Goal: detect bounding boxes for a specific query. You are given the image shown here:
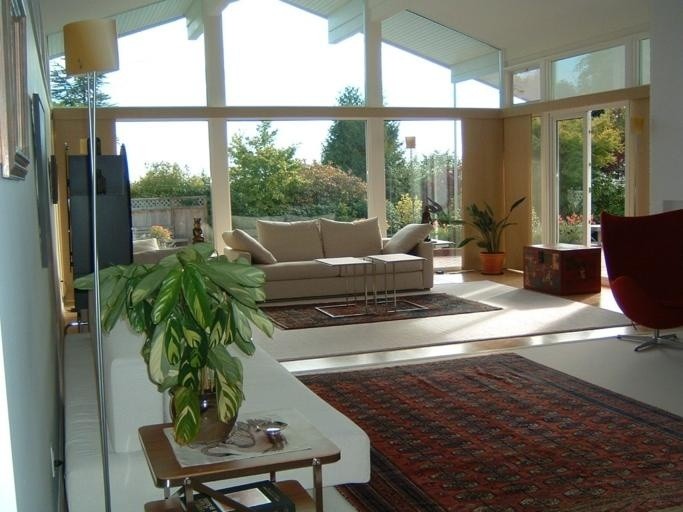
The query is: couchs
[56,266,373,510]
[221,240,435,300]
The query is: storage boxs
[523,243,602,293]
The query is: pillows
[222,228,280,265]
[132,235,157,253]
[256,216,325,267]
[382,222,434,257]
[318,216,385,257]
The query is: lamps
[404,134,420,221]
[61,17,131,512]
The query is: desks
[136,411,340,512]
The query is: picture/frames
[2,0,53,272]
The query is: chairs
[598,206,683,352]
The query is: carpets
[225,277,637,362]
[293,351,682,511]
[254,291,505,332]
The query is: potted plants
[69,240,272,445]
[428,197,533,274]
